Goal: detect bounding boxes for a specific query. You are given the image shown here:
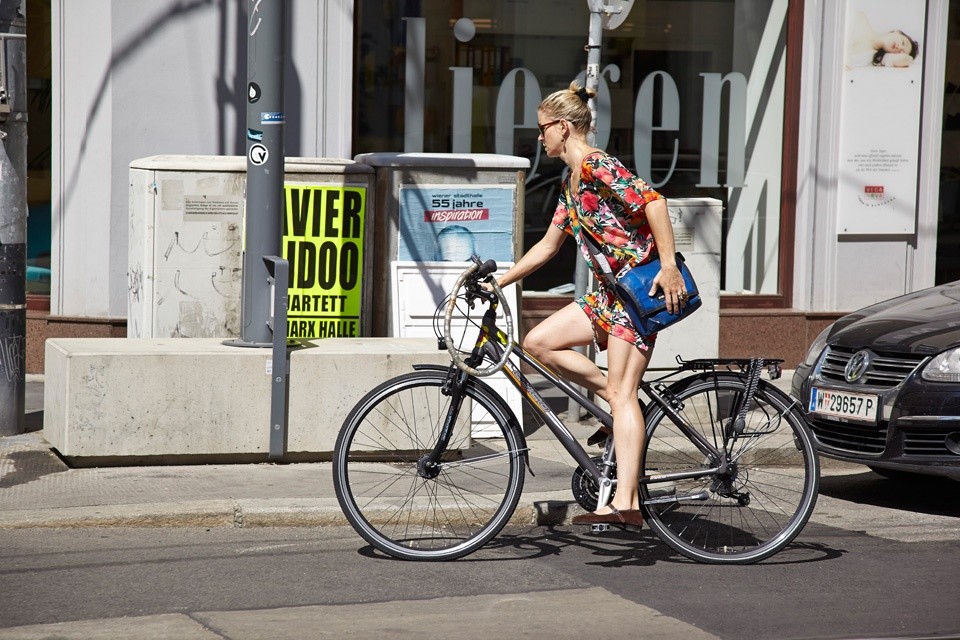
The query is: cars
[794,282,960,475]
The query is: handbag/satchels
[616,253,702,338]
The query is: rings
[679,297,684,301]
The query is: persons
[845,19,919,71]
[485,80,688,534]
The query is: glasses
[537,115,576,139]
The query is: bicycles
[331,253,822,565]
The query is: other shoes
[585,397,646,446]
[572,503,643,533]
